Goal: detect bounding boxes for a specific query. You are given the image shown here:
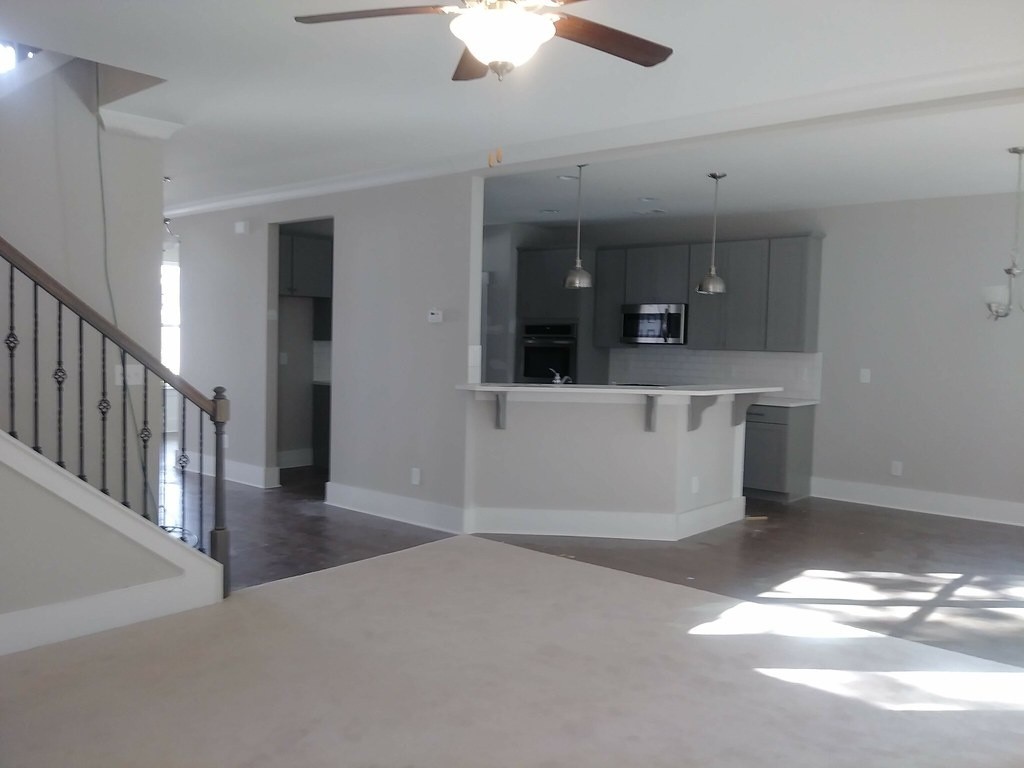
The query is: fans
[294,0,674,80]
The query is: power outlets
[410,467,421,485]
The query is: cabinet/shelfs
[279,230,333,299]
[742,406,814,505]
[593,231,826,353]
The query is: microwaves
[620,302,686,346]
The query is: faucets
[549,366,573,384]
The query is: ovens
[519,318,577,384]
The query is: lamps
[449,7,555,82]
[564,165,593,290]
[694,172,726,294]
